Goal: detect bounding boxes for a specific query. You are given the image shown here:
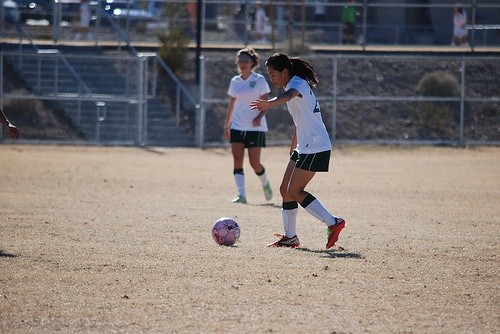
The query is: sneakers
[267,234,300,248]
[231,196,246,203]
[326,218,346,249]
[262,181,273,201]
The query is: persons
[0,106,20,140]
[452,5,470,48]
[224,48,273,204]
[340,1,360,45]
[0,0,270,47]
[250,53,346,249]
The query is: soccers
[210,216,242,245]
